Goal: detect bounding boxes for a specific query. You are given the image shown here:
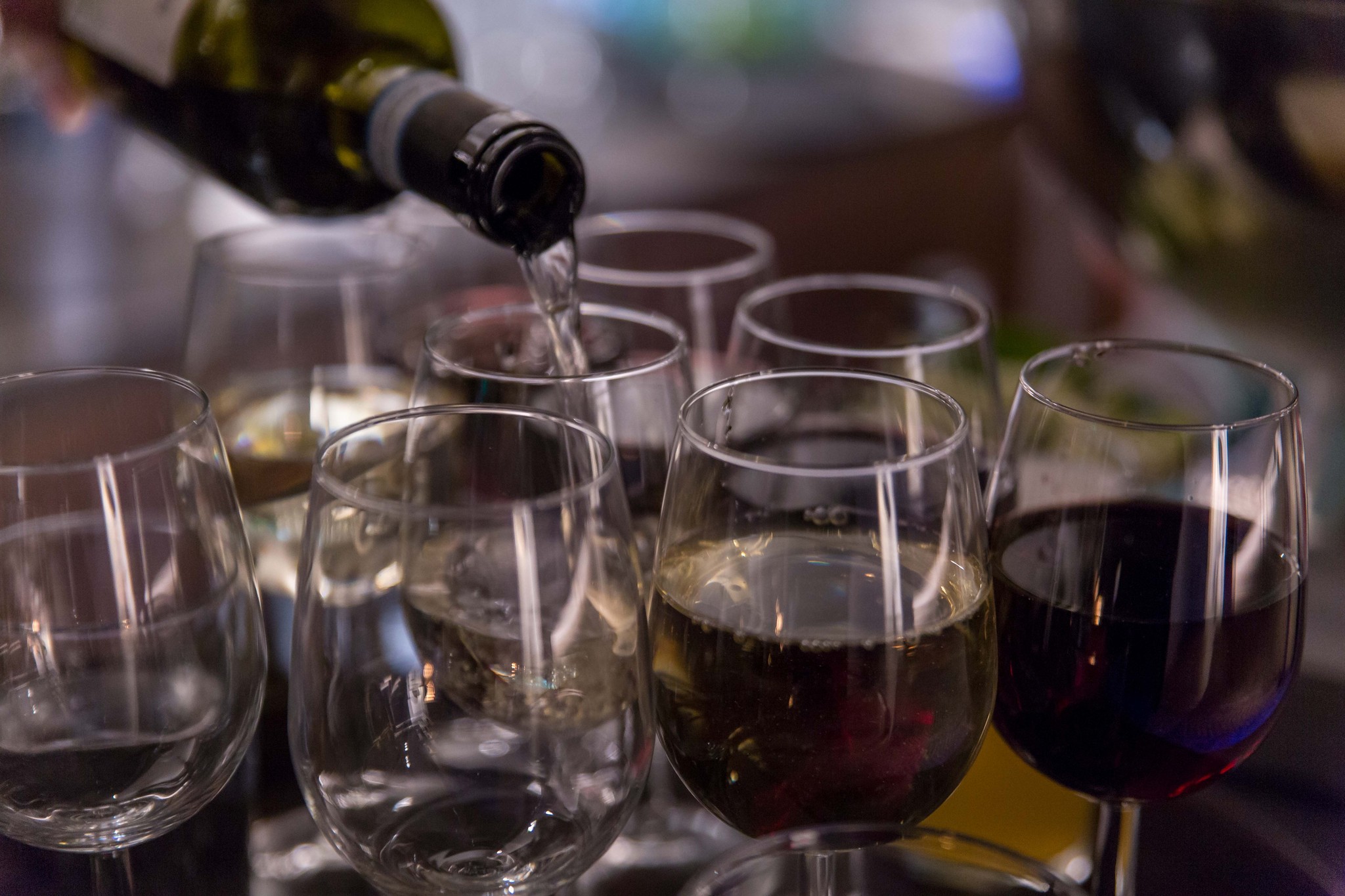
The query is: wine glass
[4,198,1309,896]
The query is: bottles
[0,2,592,251]
